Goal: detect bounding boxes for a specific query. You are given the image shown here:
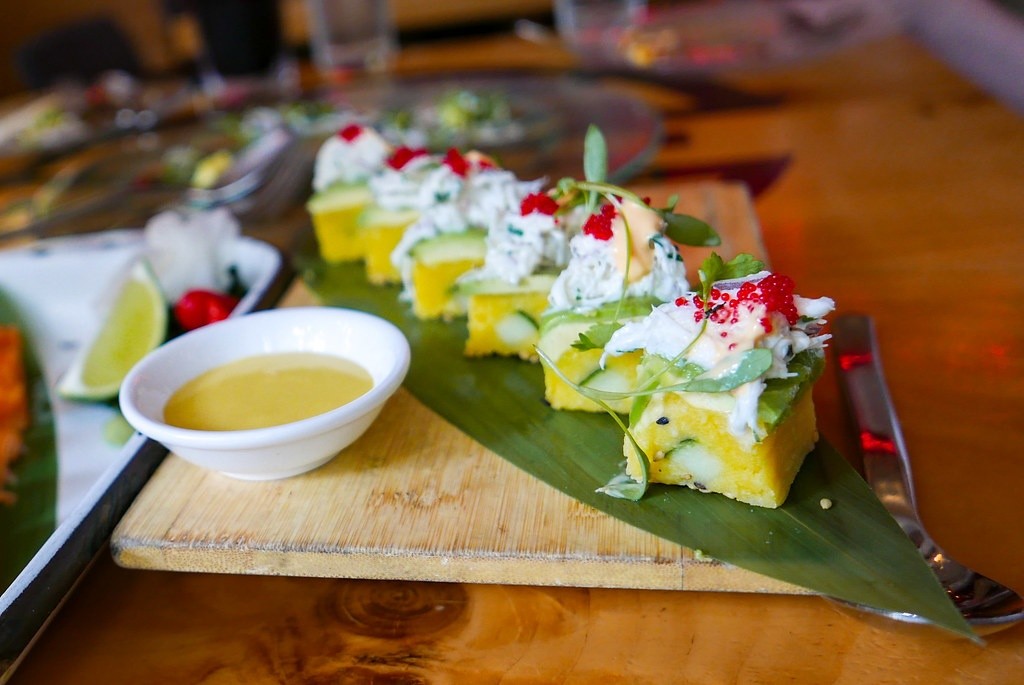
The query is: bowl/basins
[118,306,413,482]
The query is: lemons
[59,260,168,400]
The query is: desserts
[303,122,837,510]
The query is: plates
[0,227,285,685]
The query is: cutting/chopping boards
[108,178,842,596]
